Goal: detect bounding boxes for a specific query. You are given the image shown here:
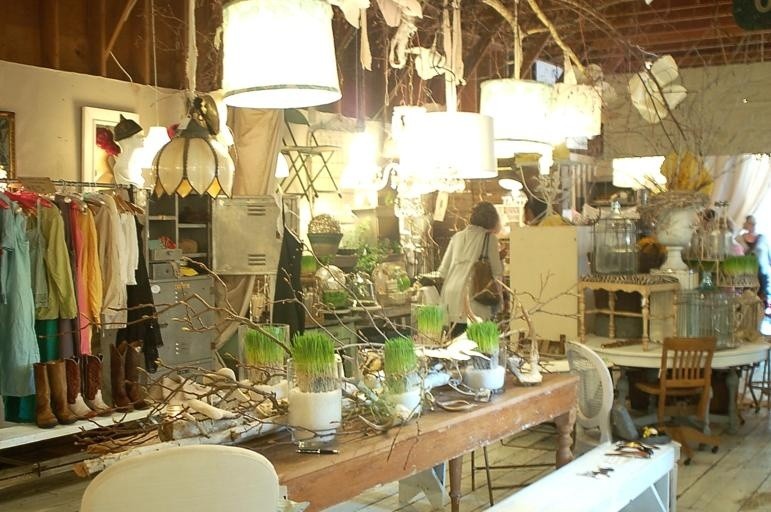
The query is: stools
[577,273,682,352]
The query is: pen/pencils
[295,449,340,454]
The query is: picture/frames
[0,110,17,180]
[79,106,141,192]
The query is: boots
[84,352,113,416]
[66,356,98,420]
[126,337,152,410]
[33,362,60,428]
[46,360,79,425]
[110,340,134,413]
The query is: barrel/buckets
[688,268,727,350]
[593,198,638,274]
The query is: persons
[436,200,504,335]
[735,214,771,318]
[114,114,145,189]
[696,207,717,236]
[522,196,547,226]
[716,216,745,255]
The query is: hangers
[1,178,146,215]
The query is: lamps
[212,1,343,110]
[131,0,238,200]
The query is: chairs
[633,334,722,466]
[564,340,614,460]
[278,111,344,203]
[78,443,280,511]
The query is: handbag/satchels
[473,261,501,306]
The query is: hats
[114,113,144,142]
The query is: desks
[234,371,583,512]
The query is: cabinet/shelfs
[126,189,280,388]
